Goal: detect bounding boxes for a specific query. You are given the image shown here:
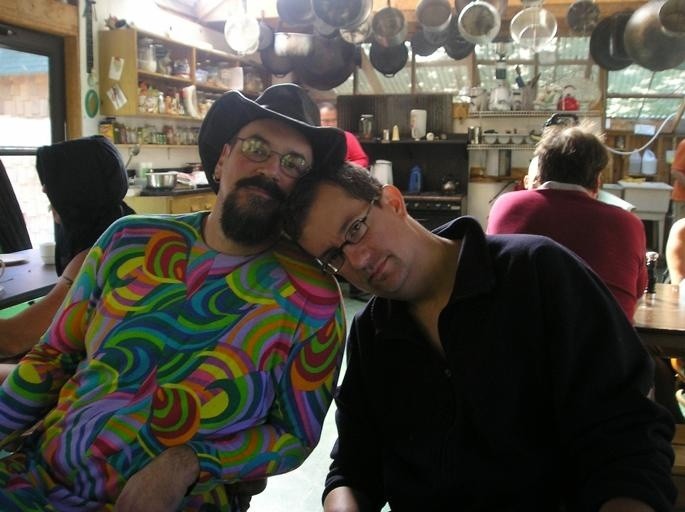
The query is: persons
[315,102,374,302]
[284,160,678,512]
[666,138,685,288]
[0,134,138,357]
[0,83,349,512]
[524,155,637,215]
[485,116,648,329]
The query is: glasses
[230,135,313,180]
[320,196,379,274]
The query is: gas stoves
[402,190,465,199]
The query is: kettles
[374,160,394,186]
[356,114,376,139]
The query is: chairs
[223,477,266,512]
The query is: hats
[197,84,346,197]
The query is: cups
[40,243,56,265]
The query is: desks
[617,180,674,257]
[0,249,62,311]
[633,281,685,356]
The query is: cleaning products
[145,162,153,173]
[136,163,146,177]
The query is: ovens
[407,209,461,231]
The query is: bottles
[511,92,522,111]
[138,38,156,72]
[629,147,641,174]
[411,109,427,141]
[107,118,121,144]
[392,125,400,141]
[409,165,424,193]
[645,252,659,293]
[137,128,143,144]
[99,122,114,144]
[383,129,389,141]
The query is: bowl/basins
[145,172,177,189]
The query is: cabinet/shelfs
[98,29,271,121]
[465,108,604,151]
[123,190,219,215]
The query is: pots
[259,9,275,52]
[416,1,452,32]
[445,16,475,60]
[261,52,294,79]
[372,0,409,46]
[509,0,558,51]
[411,30,439,56]
[295,34,353,90]
[224,0,260,52]
[370,44,409,77]
[277,0,315,23]
[590,10,634,71]
[457,0,502,45]
[624,0,685,71]
[566,1,599,35]
[312,0,372,29]
[339,15,371,44]
[274,32,316,58]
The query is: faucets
[125,153,137,170]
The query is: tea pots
[440,176,460,195]
[558,85,580,110]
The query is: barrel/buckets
[641,148,657,176]
[628,147,641,177]
